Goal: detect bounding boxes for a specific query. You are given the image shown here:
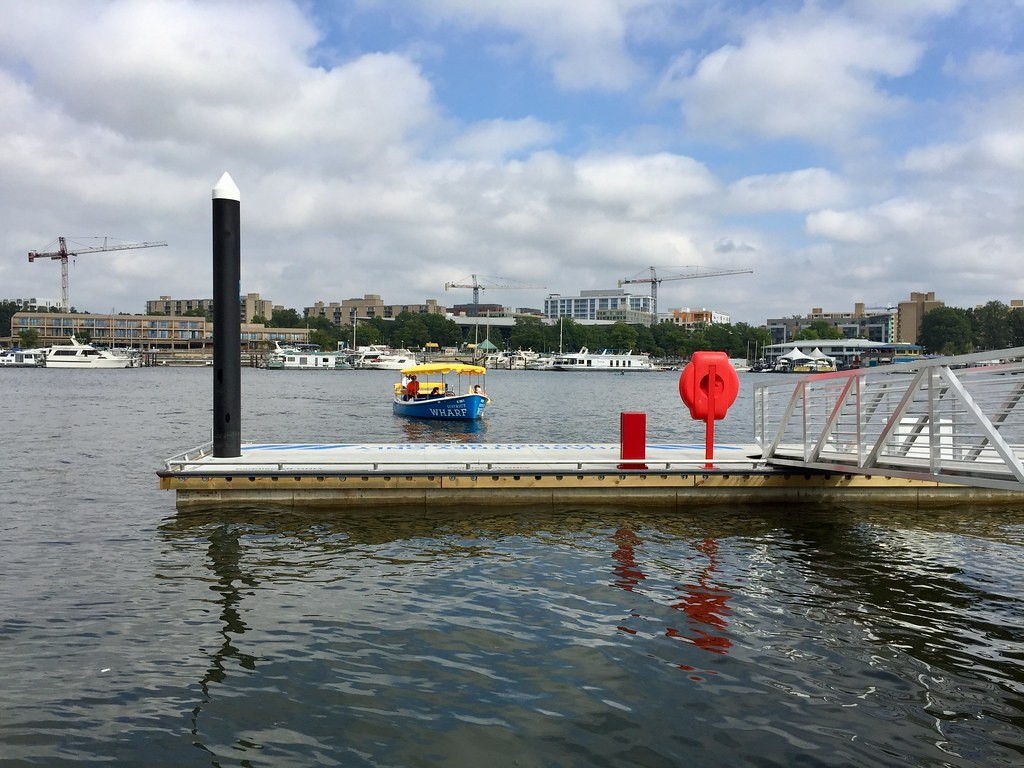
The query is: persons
[406,375,419,401]
[474,385,480,393]
[402,374,412,389]
[429,387,442,398]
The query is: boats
[393,363,491,418]
[728,359,751,372]
[370,355,417,369]
[484,346,563,371]
[860,343,929,375]
[45,335,132,368]
[553,347,667,372]
[0,347,52,368]
[105,348,144,367]
[346,347,382,370]
[256,341,355,371]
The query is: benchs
[394,382,445,401]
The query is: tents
[477,339,498,358]
[777,347,814,372]
[800,347,837,371]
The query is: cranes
[445,273,547,311]
[618,265,753,324]
[28,235,168,311]
